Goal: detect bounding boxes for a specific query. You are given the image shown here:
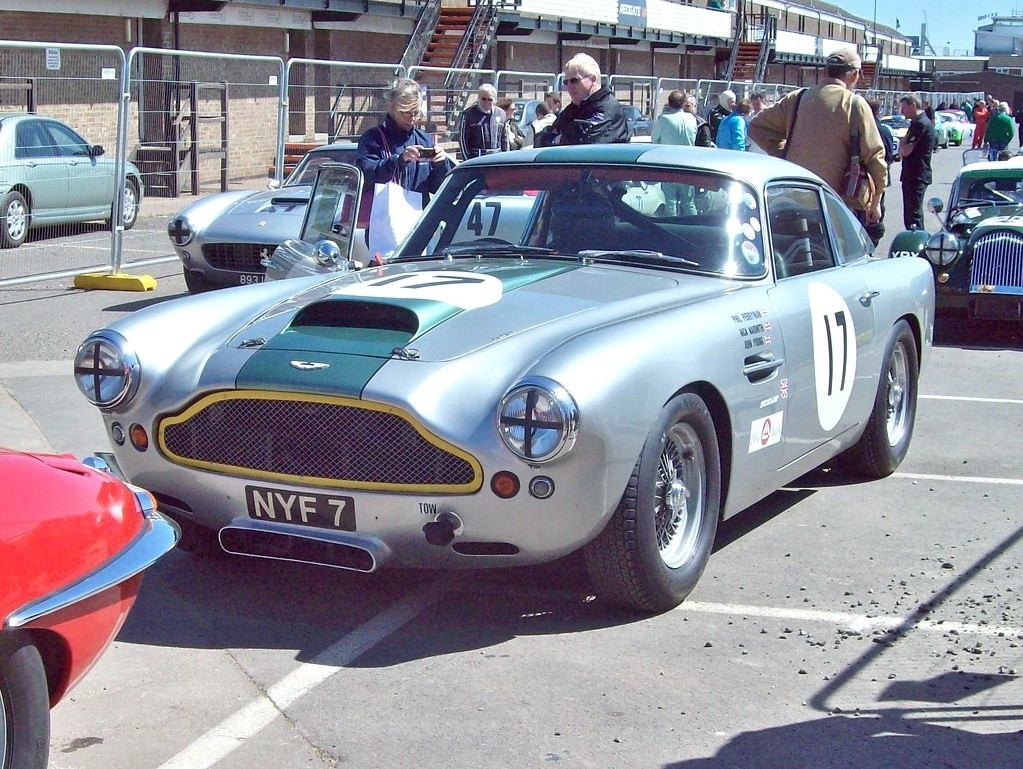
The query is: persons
[355,78,452,255]
[970,95,1015,162]
[459,83,510,160]
[532,52,630,241]
[706,91,737,146]
[704,95,720,121]
[865,98,894,223]
[899,95,933,229]
[747,93,767,155]
[522,102,561,147]
[1015,103,1023,147]
[938,98,973,119]
[546,91,563,118]
[683,93,710,147]
[748,48,888,245]
[648,89,697,216]
[924,100,940,153]
[716,99,752,151]
[494,98,526,151]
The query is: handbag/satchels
[339,128,399,228]
[368,164,423,261]
[839,163,876,211]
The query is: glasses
[564,74,593,86]
[480,96,493,102]
[506,108,516,113]
[395,108,423,119]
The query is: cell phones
[417,148,437,159]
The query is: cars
[510,99,548,140]
[888,147,1022,330]
[0,112,145,251]
[621,104,652,140]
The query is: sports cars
[72,140,939,611]
[166,138,674,298]
[880,108,973,163]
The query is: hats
[827,48,865,80]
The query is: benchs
[614,213,818,278]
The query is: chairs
[543,192,616,258]
